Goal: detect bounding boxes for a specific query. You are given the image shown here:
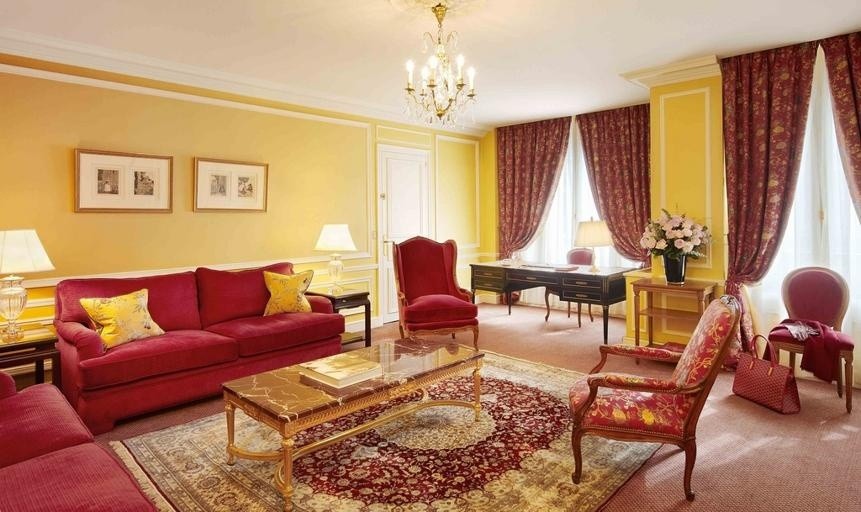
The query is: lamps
[0,229,56,343]
[575,215,614,273]
[312,224,356,292]
[390,1,478,129]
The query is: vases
[663,254,687,286]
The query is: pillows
[196,262,294,329]
[262,270,313,316]
[76,289,166,350]
[59,272,201,331]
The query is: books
[299,354,382,389]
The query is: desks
[470,264,643,345]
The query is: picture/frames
[75,148,173,214]
[194,158,269,213]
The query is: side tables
[0,326,62,392]
[630,278,717,349]
[310,290,371,347]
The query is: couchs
[0,370,160,512]
[52,281,345,436]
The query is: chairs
[570,295,742,501]
[545,247,595,328]
[768,266,854,414]
[393,236,479,350]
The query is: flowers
[640,208,712,259]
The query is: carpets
[108,343,663,512]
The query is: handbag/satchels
[732,335,802,415]
